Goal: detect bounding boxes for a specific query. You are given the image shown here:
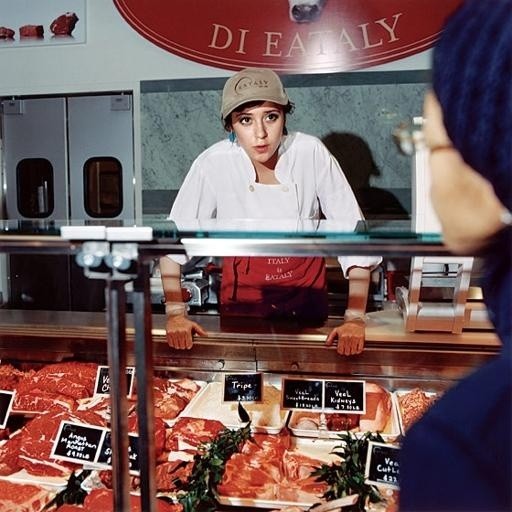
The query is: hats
[431,1,512,212]
[222,68,288,118]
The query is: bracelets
[343,309,370,325]
[164,301,191,316]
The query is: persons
[396,0,511,512]
[159,68,384,357]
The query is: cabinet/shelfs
[1,227,504,512]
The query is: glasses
[392,117,456,157]
